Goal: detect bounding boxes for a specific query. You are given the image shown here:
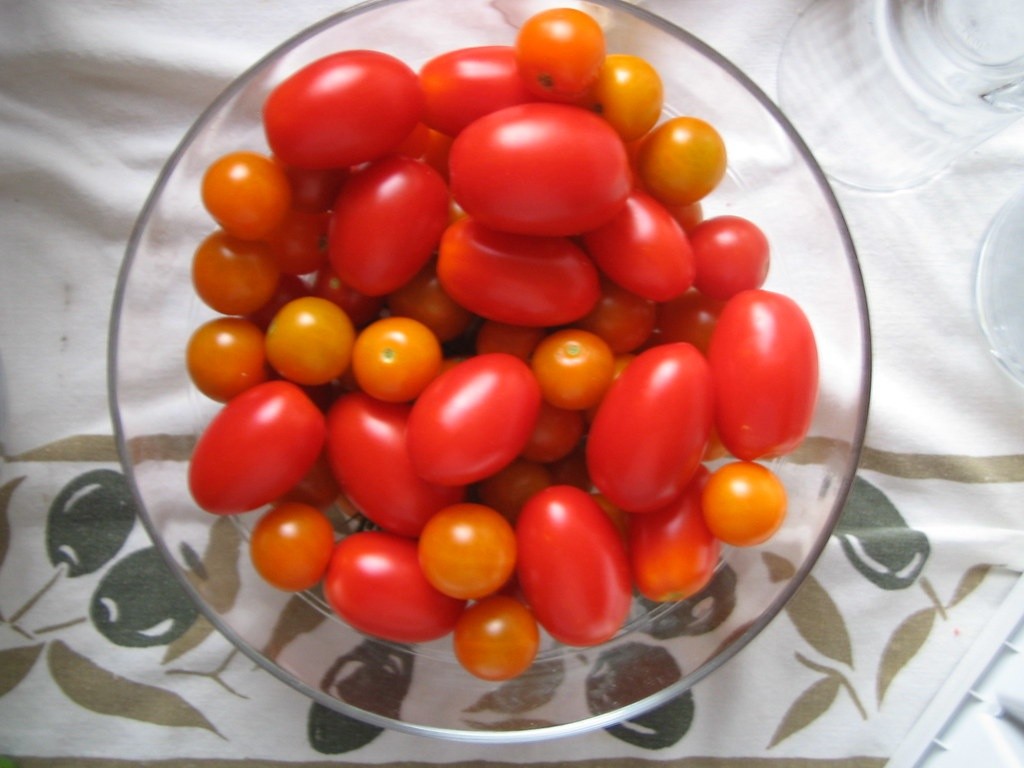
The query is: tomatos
[187,8,818,682]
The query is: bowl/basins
[108,0,873,745]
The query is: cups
[978,192,1024,386]
[780,0,1024,193]
